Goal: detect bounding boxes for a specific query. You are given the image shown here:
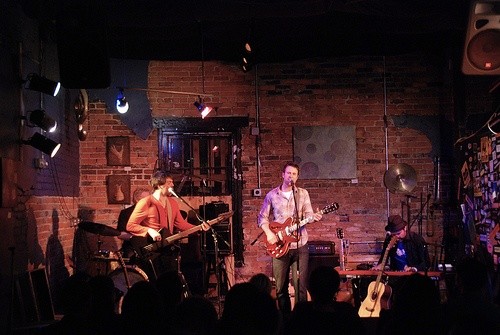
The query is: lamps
[17,72,61,97]
[12,108,57,133]
[194,95,212,119]
[15,132,61,157]
[241,41,256,73]
[116,91,129,114]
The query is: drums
[108,264,150,294]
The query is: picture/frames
[106,136,130,166]
[107,175,131,204]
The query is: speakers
[199,202,230,223]
[308,242,335,255]
[201,225,232,255]
[298,254,342,273]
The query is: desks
[94,255,130,276]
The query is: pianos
[335,266,444,300]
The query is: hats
[385,215,407,232]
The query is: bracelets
[129,235,134,241]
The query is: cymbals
[384,163,418,194]
[77,221,122,236]
[88,255,130,263]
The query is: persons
[117,188,153,258]
[376,213,430,271]
[126,169,211,285]
[258,162,322,323]
[52,270,441,335]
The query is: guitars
[263,202,339,259]
[357,233,401,317]
[122,211,235,275]
[335,226,355,307]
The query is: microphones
[289,177,299,193]
[168,186,183,201]
